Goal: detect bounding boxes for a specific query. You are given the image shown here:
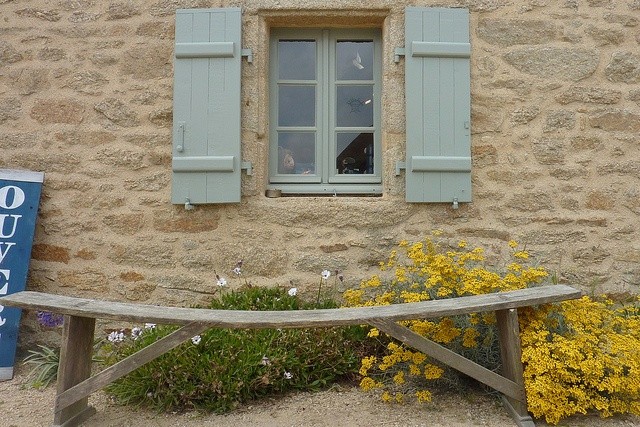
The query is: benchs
[0,283,582,427]
